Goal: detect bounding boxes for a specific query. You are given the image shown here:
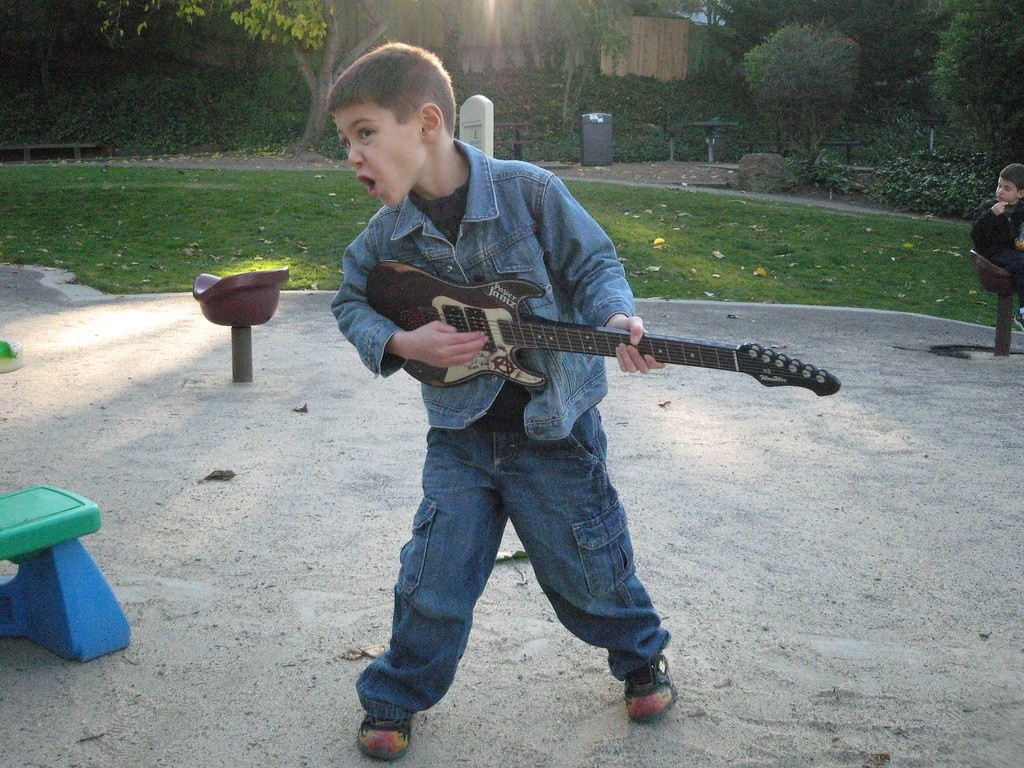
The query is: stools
[0,485,131,663]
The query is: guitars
[363,256,843,397]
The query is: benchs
[765,139,863,167]
[493,121,535,160]
[0,141,102,163]
[493,139,536,161]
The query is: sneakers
[357,712,412,759]
[624,645,678,724]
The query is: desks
[658,119,739,162]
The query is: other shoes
[1014,310,1024,331]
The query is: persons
[324,40,682,763]
[969,161,1024,333]
